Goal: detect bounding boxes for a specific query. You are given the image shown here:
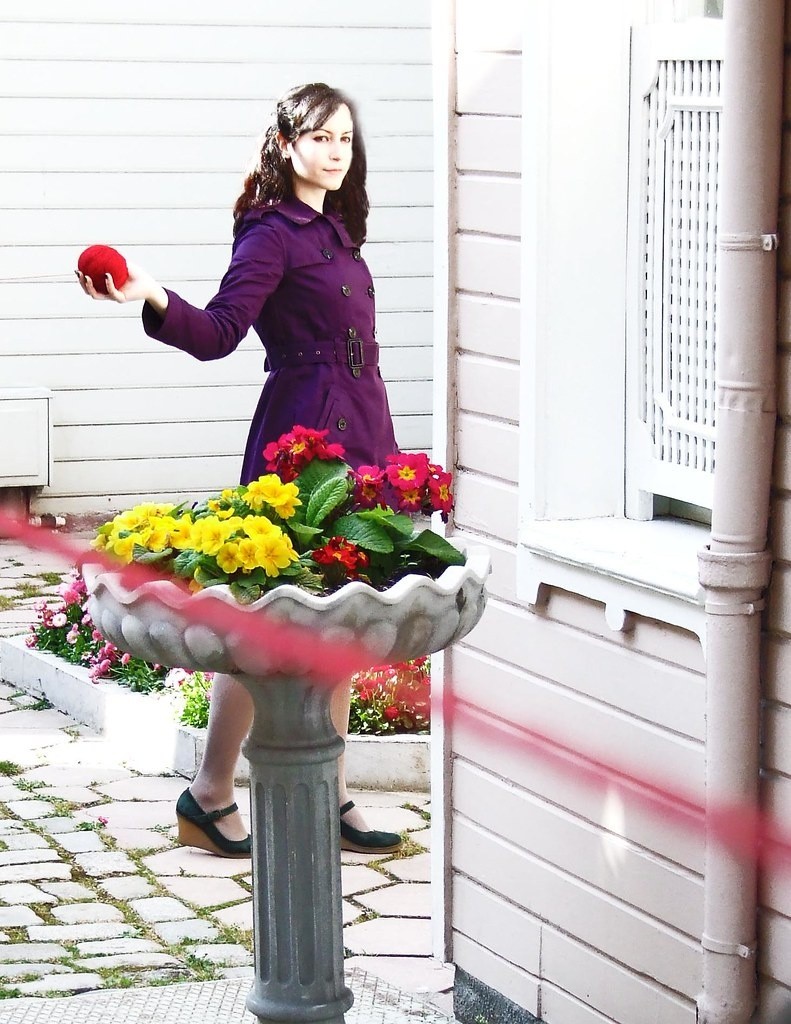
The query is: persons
[69,83,402,855]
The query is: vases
[83,563,486,679]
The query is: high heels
[340,800,403,854]
[175,788,253,857]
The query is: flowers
[86,427,468,606]
